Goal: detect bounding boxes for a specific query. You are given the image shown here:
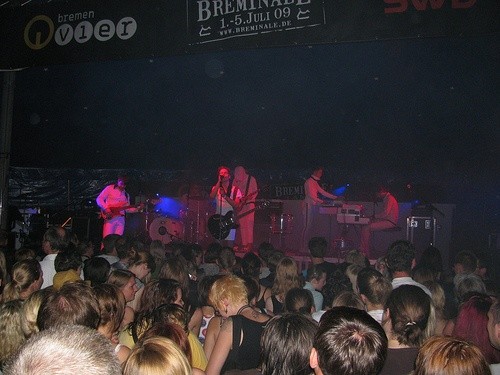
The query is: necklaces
[239,306,251,316]
[237,305,248,315]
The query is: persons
[307,236,338,309]
[96,175,143,250]
[359,183,398,258]
[0,225,209,375]
[191,242,500,375]
[209,166,258,251]
[298,165,345,254]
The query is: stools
[383,226,401,244]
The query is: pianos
[318,201,369,258]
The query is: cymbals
[189,195,207,200]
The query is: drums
[180,208,194,220]
[149,216,183,245]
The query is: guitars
[208,200,271,240]
[101,205,134,220]
[240,183,269,202]
